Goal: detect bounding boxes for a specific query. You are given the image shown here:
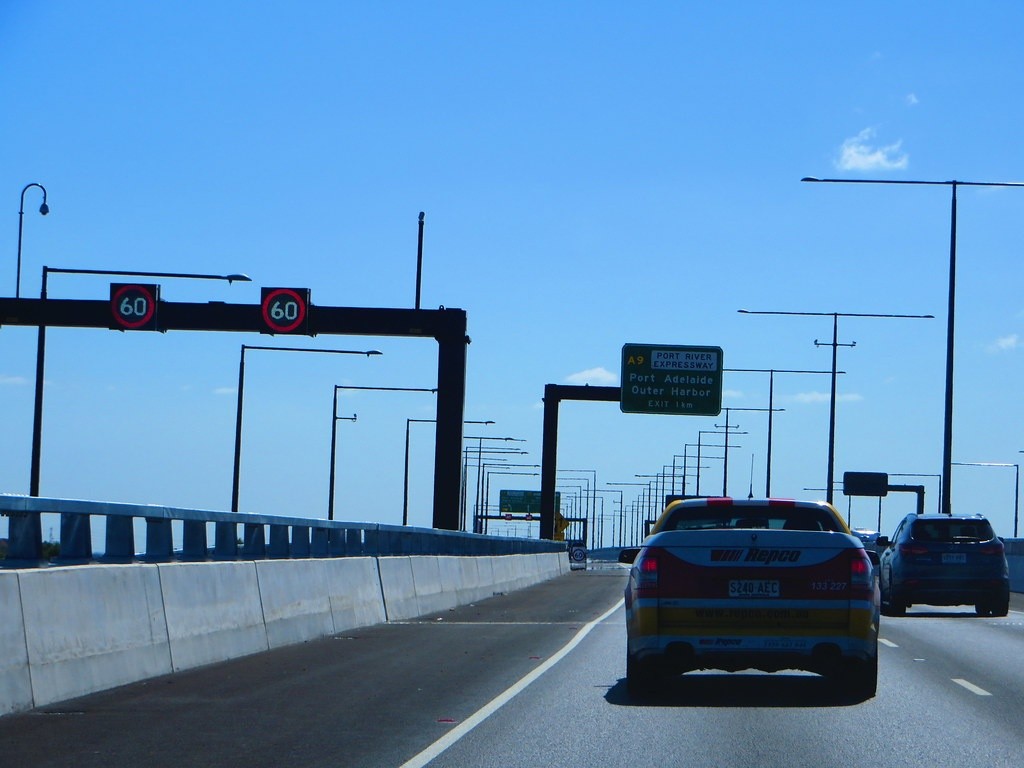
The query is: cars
[850,527,888,575]
[614,496,881,700]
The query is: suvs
[876,513,1010,618]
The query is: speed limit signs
[257,285,313,336]
[106,282,162,332]
[572,549,584,561]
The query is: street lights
[402,417,496,525]
[566,443,743,564]
[695,430,749,498]
[460,436,541,535]
[721,407,789,498]
[30,260,255,499]
[800,175,1024,514]
[722,368,847,498]
[230,343,384,513]
[14,180,51,300]
[736,308,936,505]
[802,486,851,529]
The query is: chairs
[960,527,976,537]
[783,518,821,531]
